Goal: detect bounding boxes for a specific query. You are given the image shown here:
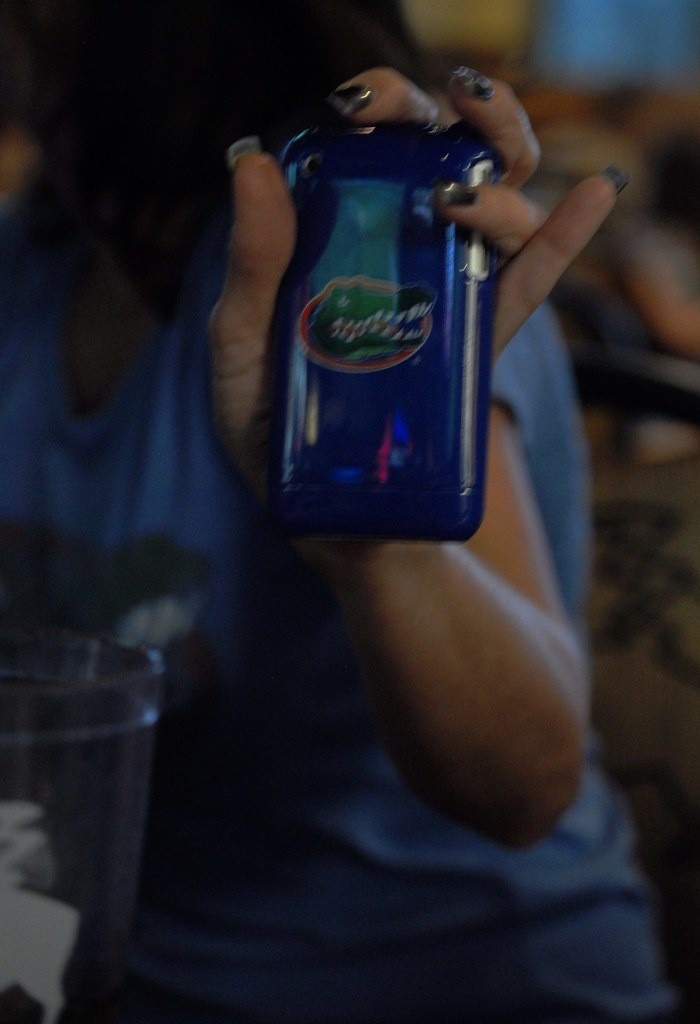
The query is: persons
[0,1,698,1024]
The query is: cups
[0,627,159,973]
